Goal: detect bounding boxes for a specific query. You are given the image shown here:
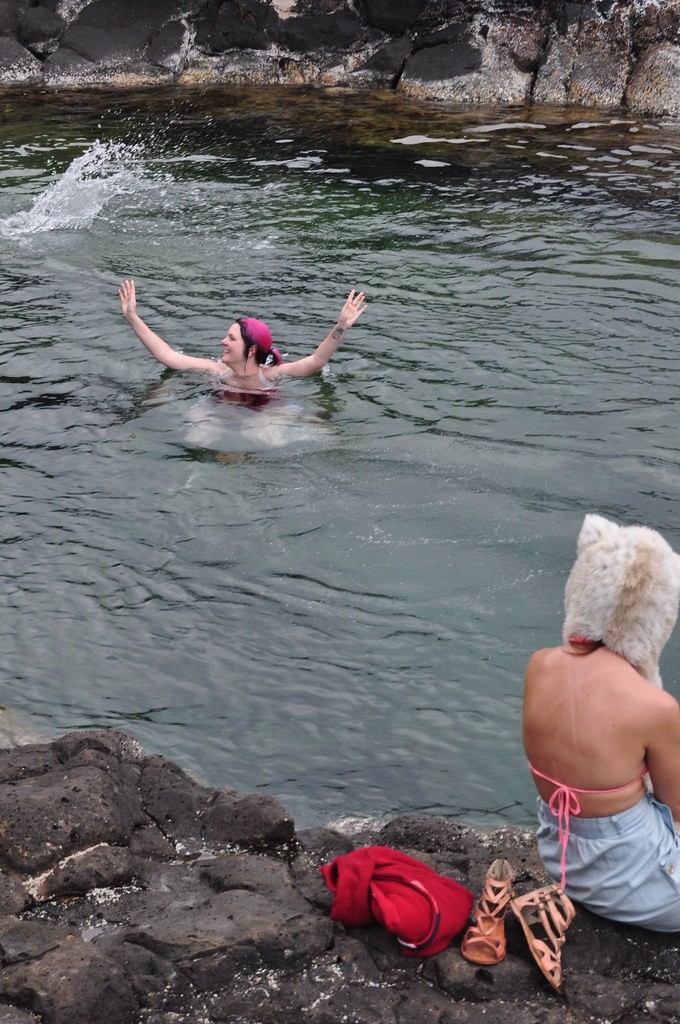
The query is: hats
[560,512,680,687]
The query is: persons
[522,514,679,933]
[118,279,367,389]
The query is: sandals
[461,860,513,966]
[508,881,576,994]
